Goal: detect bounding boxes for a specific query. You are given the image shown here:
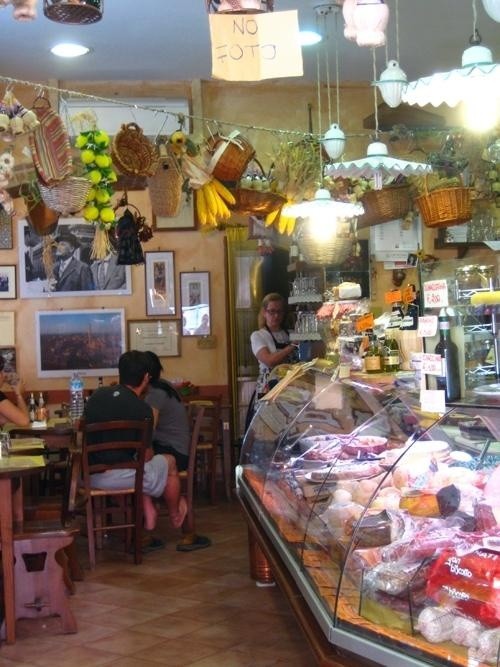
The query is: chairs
[9,432,81,635]
[81,417,152,577]
[188,392,220,505]
[145,403,205,533]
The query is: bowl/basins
[345,507,406,550]
[300,435,341,455]
[398,489,452,519]
[346,435,387,455]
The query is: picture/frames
[0,265,17,300]
[35,308,126,380]
[0,311,16,346]
[145,250,176,316]
[180,271,212,336]
[17,218,133,298]
[0,349,16,374]
[127,318,181,358]
[152,190,197,231]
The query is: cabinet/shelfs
[235,359,500,667]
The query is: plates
[305,465,382,484]
[408,440,453,463]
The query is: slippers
[176,534,212,552]
[135,537,165,553]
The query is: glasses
[265,309,285,315]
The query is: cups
[295,310,319,333]
[292,275,344,294]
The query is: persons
[43,233,93,289]
[0,355,30,534]
[74,350,188,531]
[140,351,190,472]
[244,292,298,436]
[90,250,126,289]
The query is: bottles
[459,266,480,289]
[309,310,318,334]
[400,284,420,329]
[70,372,85,419]
[383,330,391,372]
[482,340,496,380]
[435,316,461,402]
[99,375,103,387]
[389,329,401,372]
[478,265,489,288]
[455,268,464,290]
[301,315,307,333]
[38,392,46,421]
[305,311,311,333]
[388,289,404,328]
[297,311,303,333]
[365,313,374,341]
[28,392,38,422]
[365,335,385,373]
[488,264,497,289]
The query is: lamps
[325,44,434,191]
[316,4,347,161]
[281,3,365,239]
[402,0,500,136]
[370,0,409,108]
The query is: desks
[0,437,51,645]
[10,422,82,522]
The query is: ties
[58,262,65,278]
[98,260,108,287]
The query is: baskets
[296,215,355,267]
[354,182,413,232]
[36,176,92,215]
[28,98,73,185]
[416,166,474,227]
[230,159,288,216]
[149,154,183,219]
[112,121,160,178]
[206,129,256,183]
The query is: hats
[54,232,81,249]
